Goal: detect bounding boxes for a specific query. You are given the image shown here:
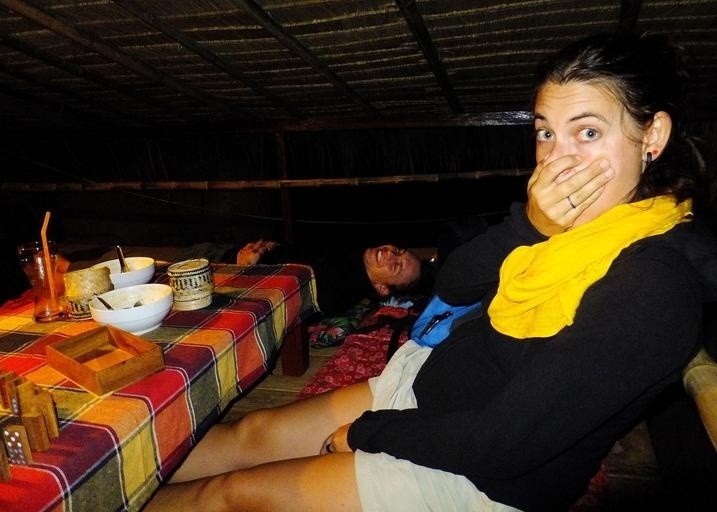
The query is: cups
[70,271,111,322]
[166,256,213,313]
[18,240,66,325]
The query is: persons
[58,230,436,323]
[139,38,707,512]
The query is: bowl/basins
[97,257,154,293]
[89,282,175,337]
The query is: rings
[567,196,576,208]
